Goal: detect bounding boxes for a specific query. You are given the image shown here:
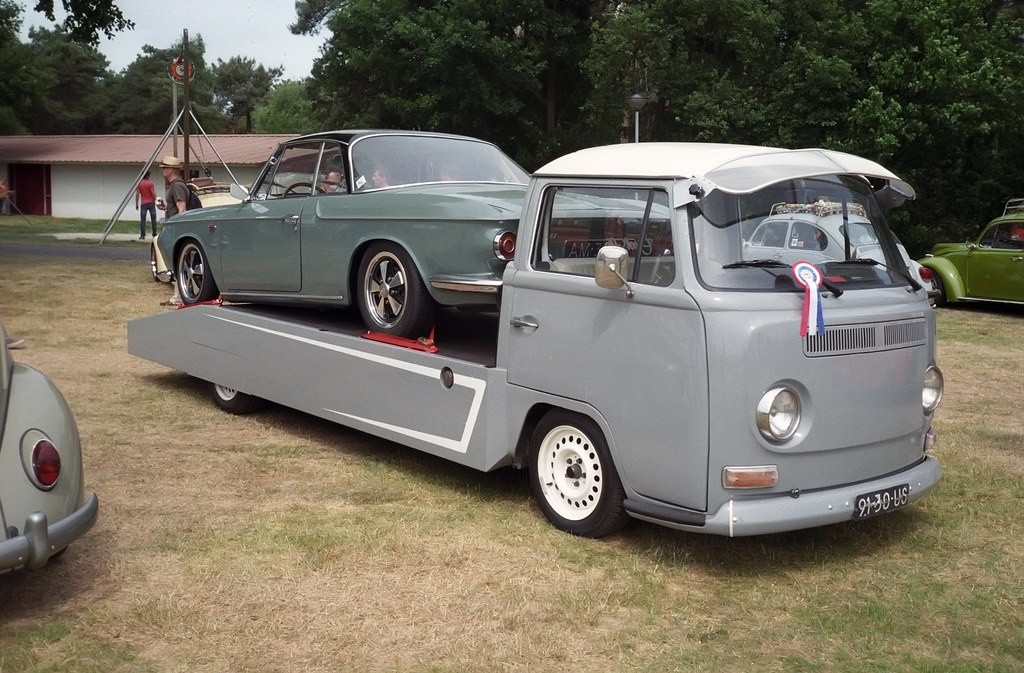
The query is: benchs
[553,257,675,288]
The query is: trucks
[125,141,945,538]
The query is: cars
[150,176,277,284]
[156,128,672,341]
[916,198,1024,310]
[0,326,102,591]
[740,202,942,311]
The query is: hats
[159,155,182,168]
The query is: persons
[160,155,189,306]
[135,170,157,240]
[0,175,15,215]
[370,167,390,188]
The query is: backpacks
[171,178,202,211]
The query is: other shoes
[152,233,157,237]
[140,235,144,239]
[160,300,175,306]
